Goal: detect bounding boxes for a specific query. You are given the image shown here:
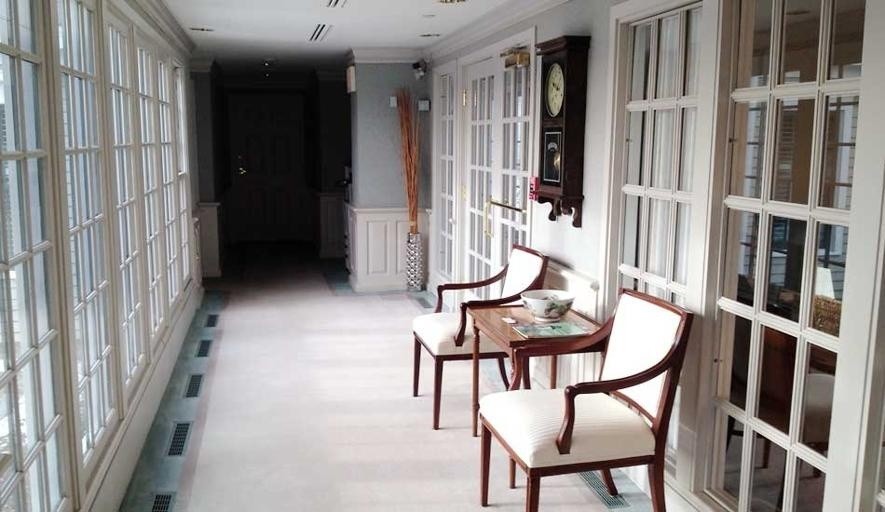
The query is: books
[512,321,591,338]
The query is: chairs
[412,245,549,430]
[477,288,695,511]
[725,295,835,512]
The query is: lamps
[412,59,428,81]
[815,266,836,299]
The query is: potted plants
[398,86,424,293]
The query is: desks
[467,304,605,489]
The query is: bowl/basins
[521,288,576,321]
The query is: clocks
[529,35,592,228]
